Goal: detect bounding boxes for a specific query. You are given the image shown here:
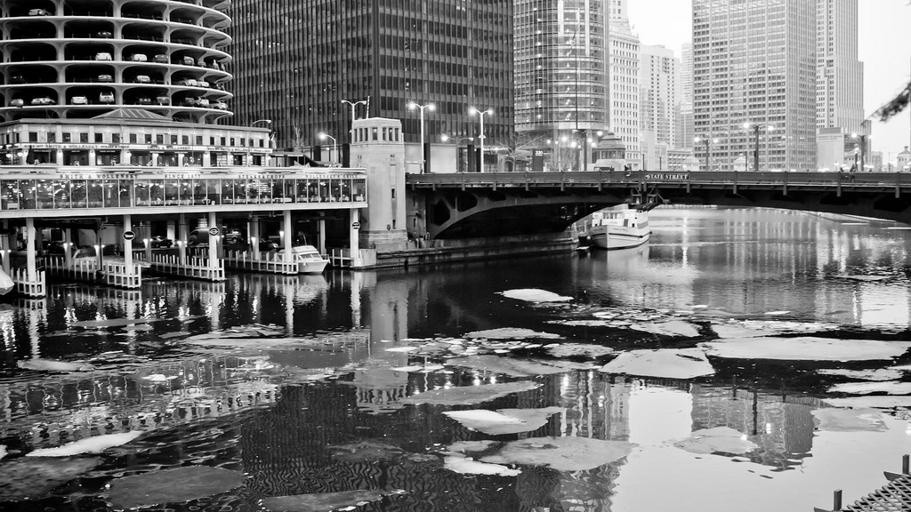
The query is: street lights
[639,151,648,170]
[740,150,753,173]
[441,133,474,172]
[244,118,271,168]
[468,106,493,173]
[409,102,434,173]
[319,131,337,167]
[743,122,775,173]
[573,129,603,172]
[340,99,368,120]
[695,135,719,172]
[656,152,665,171]
[850,132,874,173]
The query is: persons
[624,165,629,171]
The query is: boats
[269,230,331,274]
[588,208,654,251]
[0,266,16,298]
[61,244,152,283]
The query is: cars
[144,234,173,248]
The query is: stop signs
[123,231,136,241]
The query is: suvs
[226,228,242,243]
